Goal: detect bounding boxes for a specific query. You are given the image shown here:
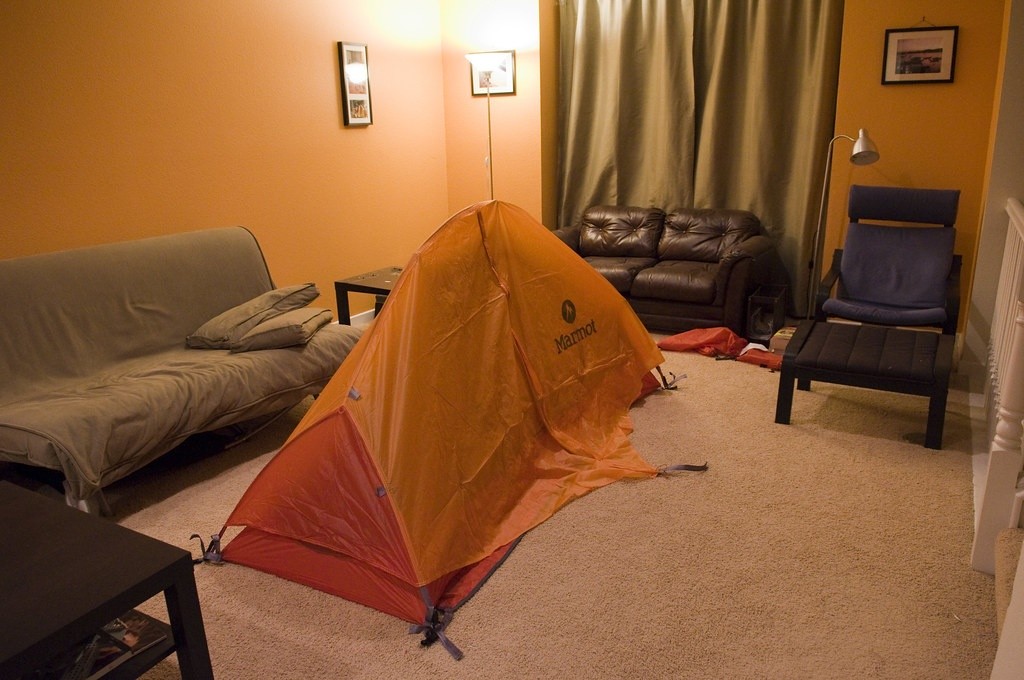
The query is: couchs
[549,206,779,340]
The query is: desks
[335,266,403,325]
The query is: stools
[773,318,953,450]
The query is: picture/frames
[471,50,516,96]
[337,42,373,127]
[882,26,959,85]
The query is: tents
[199,198,677,643]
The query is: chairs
[794,182,963,391]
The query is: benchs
[0,225,368,517]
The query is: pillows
[837,222,957,309]
[186,282,320,349]
[230,307,333,353]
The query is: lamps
[807,128,880,318]
[469,54,506,201]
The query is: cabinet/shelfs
[0,480,213,680]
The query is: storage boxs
[769,327,796,354]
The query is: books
[100,609,167,657]
[54,629,132,680]
